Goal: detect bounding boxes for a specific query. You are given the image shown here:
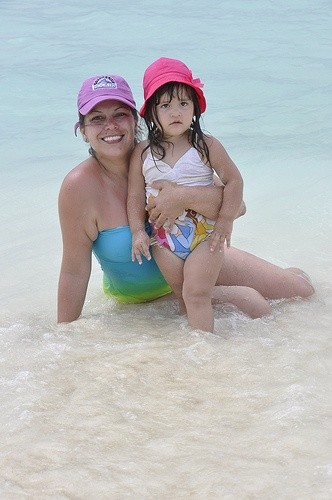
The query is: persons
[127,55,243,334]
[56,73,316,324]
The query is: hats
[138,57,206,118]
[75,74,137,137]
